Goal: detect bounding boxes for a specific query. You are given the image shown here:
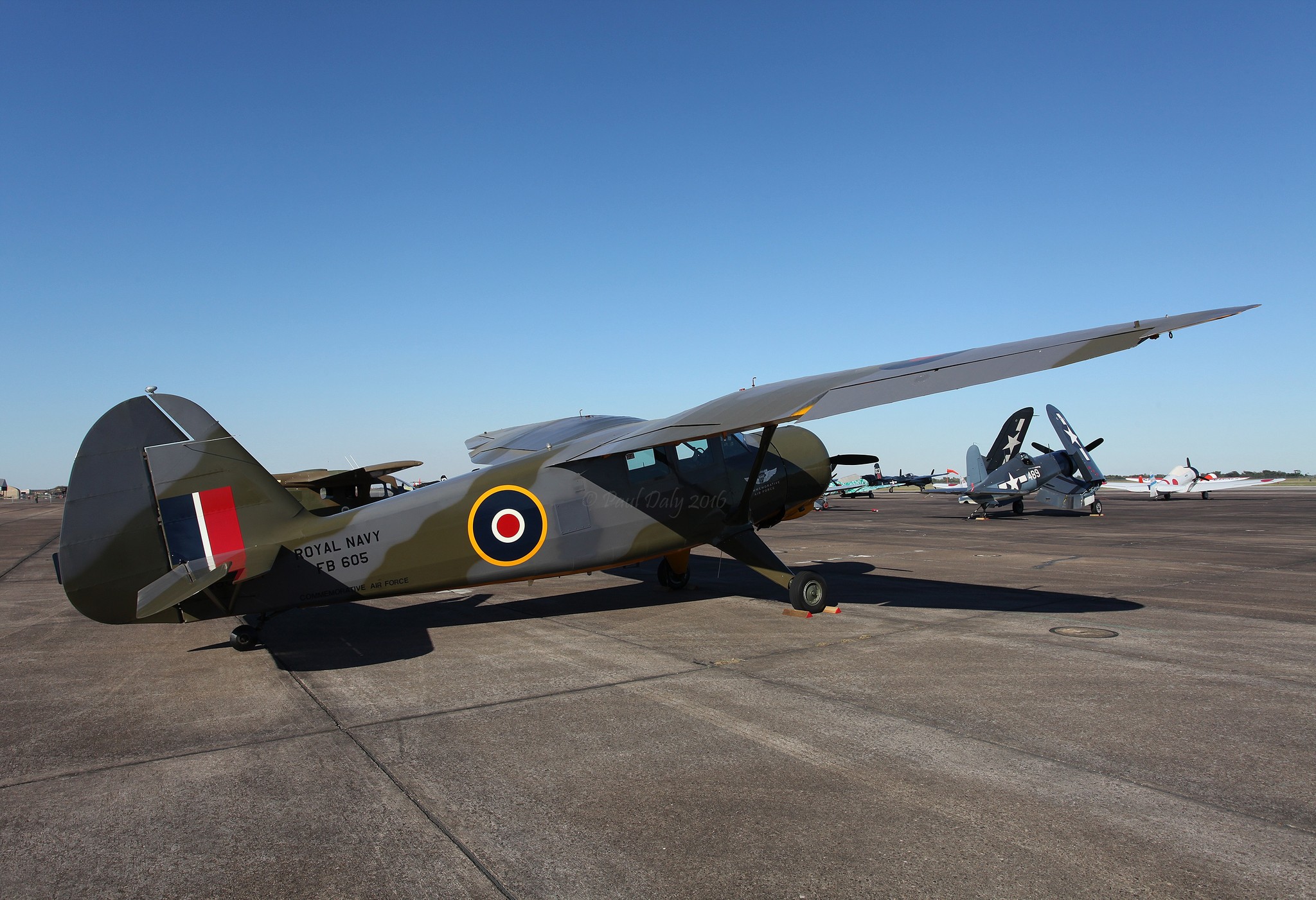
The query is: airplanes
[957,402,1106,517]
[50,294,1264,662]
[919,477,987,506]
[1098,456,1286,499]
[821,462,951,502]
